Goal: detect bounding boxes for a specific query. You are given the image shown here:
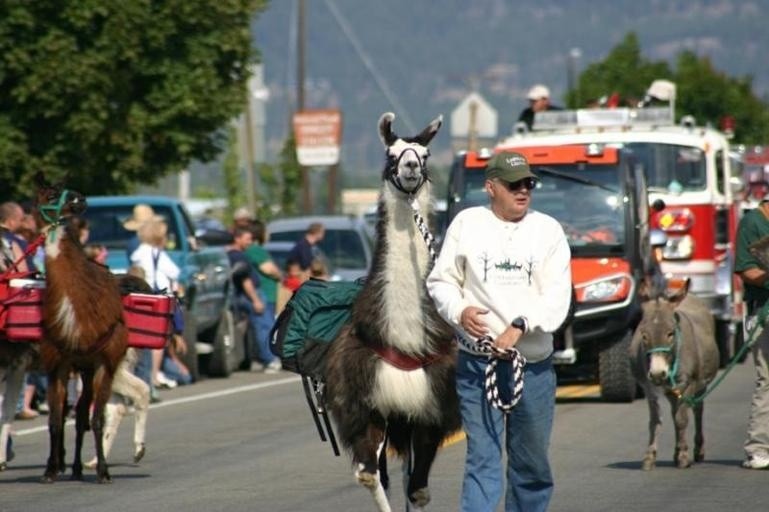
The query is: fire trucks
[495,76,768,367]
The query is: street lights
[242,84,271,210]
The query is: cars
[247,195,450,390]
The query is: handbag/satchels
[268,275,373,381]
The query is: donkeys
[627,276,720,473]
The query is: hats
[233,209,257,220]
[122,204,167,231]
[488,153,540,184]
[525,84,549,101]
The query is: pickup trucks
[78,195,244,388]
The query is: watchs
[511,316,525,334]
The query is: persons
[732,188,768,468]
[518,85,569,136]
[225,205,343,374]
[1,198,200,458]
[425,148,575,510]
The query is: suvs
[446,141,667,404]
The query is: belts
[748,299,765,316]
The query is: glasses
[495,176,537,191]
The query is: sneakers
[741,449,768,470]
[14,404,40,420]
[250,357,282,374]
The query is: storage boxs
[121,292,176,348]
[6,279,46,343]
